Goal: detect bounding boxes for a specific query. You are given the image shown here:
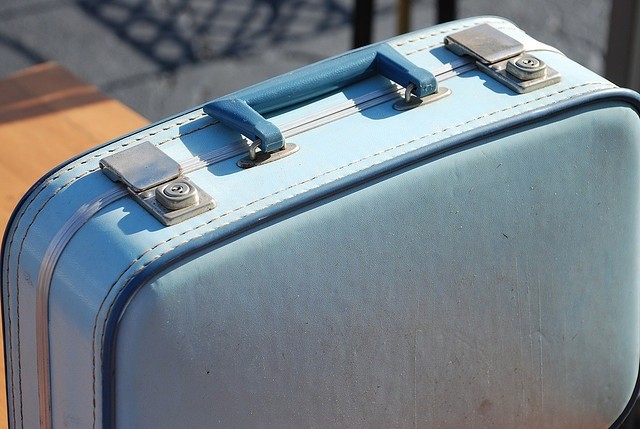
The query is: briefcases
[1,14,639,427]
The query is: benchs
[0,59,153,429]
